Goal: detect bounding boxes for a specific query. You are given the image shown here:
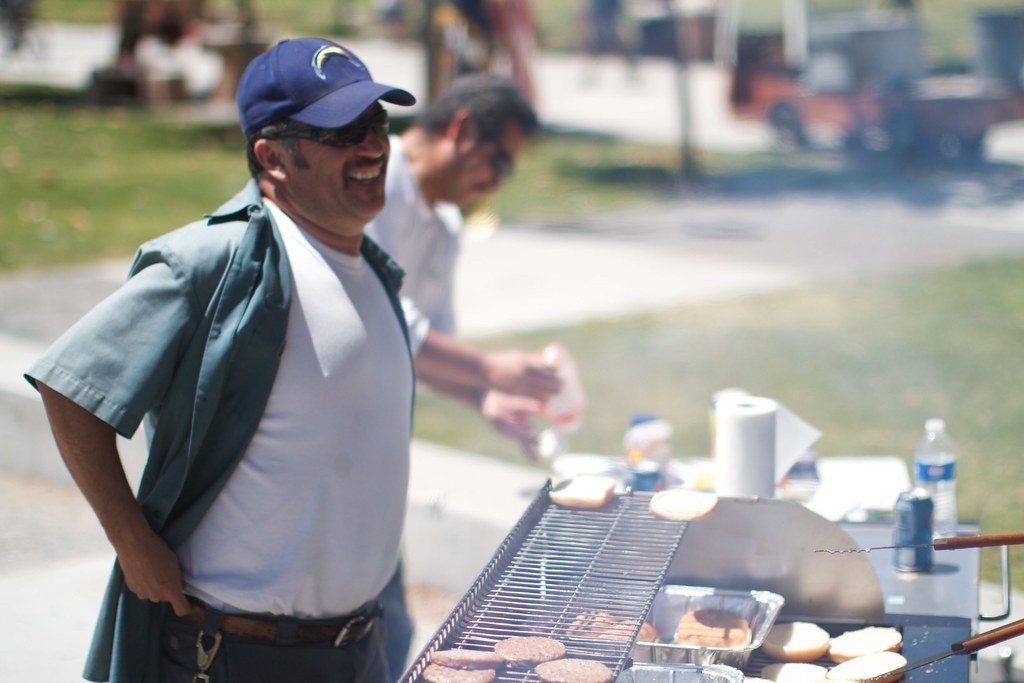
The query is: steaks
[421,636,613,683]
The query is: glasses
[251,109,391,147]
[484,133,515,179]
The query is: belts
[185,602,384,649]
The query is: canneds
[891,488,935,573]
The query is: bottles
[915,418,957,540]
[628,415,666,493]
[543,346,586,434]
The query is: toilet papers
[716,395,823,501]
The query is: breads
[546,476,615,508]
[648,489,719,521]
[743,621,907,683]
[566,606,752,650]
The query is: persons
[21,38,415,683]
[365,72,566,457]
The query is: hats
[236,36,416,137]
